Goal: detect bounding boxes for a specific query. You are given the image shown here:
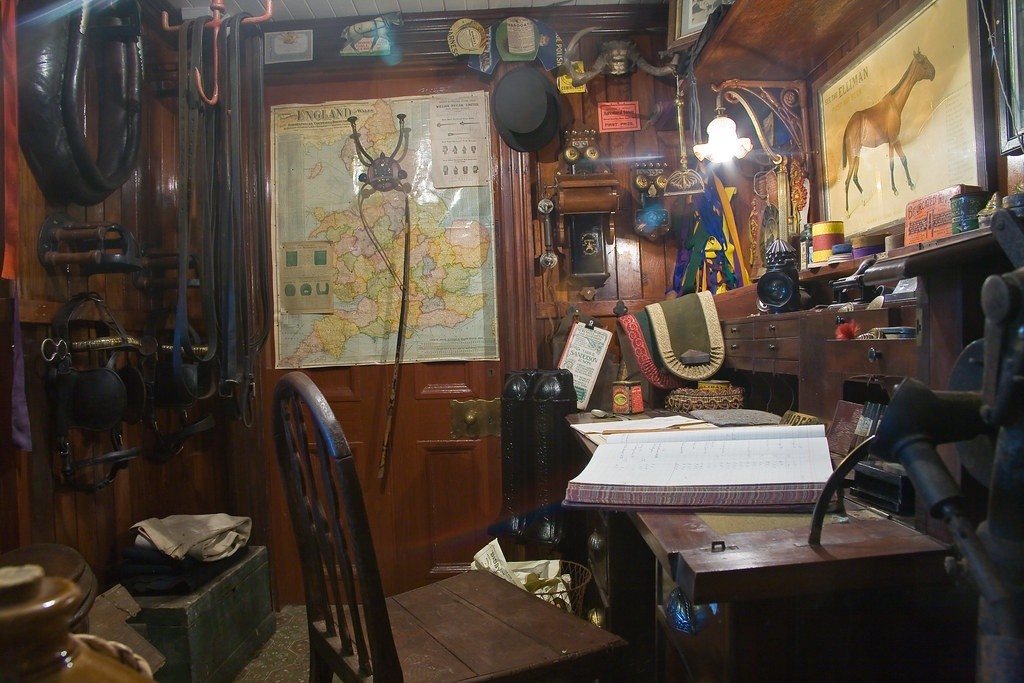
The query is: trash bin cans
[506,559,591,617]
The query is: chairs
[268,372,634,683]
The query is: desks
[563,409,960,683]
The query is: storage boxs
[903,185,980,246]
[120,544,276,683]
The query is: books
[570,415,719,447]
[560,425,843,514]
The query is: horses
[843,45,935,220]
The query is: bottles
[800,223,812,270]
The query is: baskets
[531,560,592,618]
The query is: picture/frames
[667,0,735,52]
[993,0,1024,156]
[812,0,994,243]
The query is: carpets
[236,604,363,683]
[615,290,725,408]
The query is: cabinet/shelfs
[714,227,994,542]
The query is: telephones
[539,119,619,301]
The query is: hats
[491,68,560,152]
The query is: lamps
[693,78,814,177]
